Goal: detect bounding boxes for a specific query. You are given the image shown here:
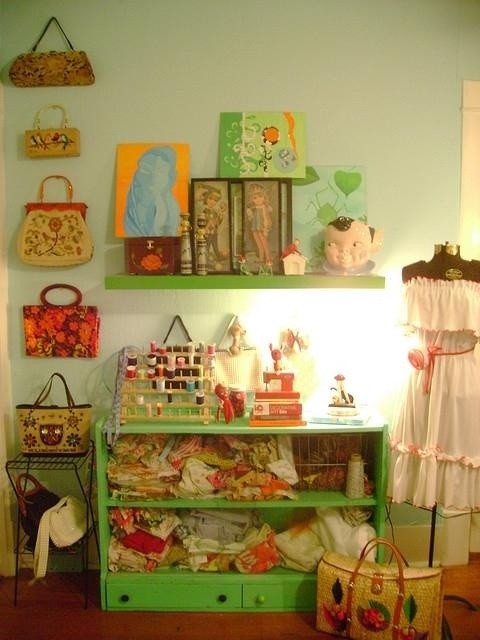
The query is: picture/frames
[189,173,293,275]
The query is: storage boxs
[124,235,182,276]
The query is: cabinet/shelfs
[95,412,389,613]
[5,438,100,610]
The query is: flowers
[37,217,63,257]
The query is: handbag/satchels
[16,474,66,536]
[16,176,94,267]
[23,284,100,358]
[50,495,96,548]
[24,104,80,158]
[9,17,95,88]
[16,373,92,457]
[316,537,448,640]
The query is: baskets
[292,435,370,490]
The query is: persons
[323,216,375,276]
[198,183,229,262]
[389,244,480,511]
[247,185,274,264]
